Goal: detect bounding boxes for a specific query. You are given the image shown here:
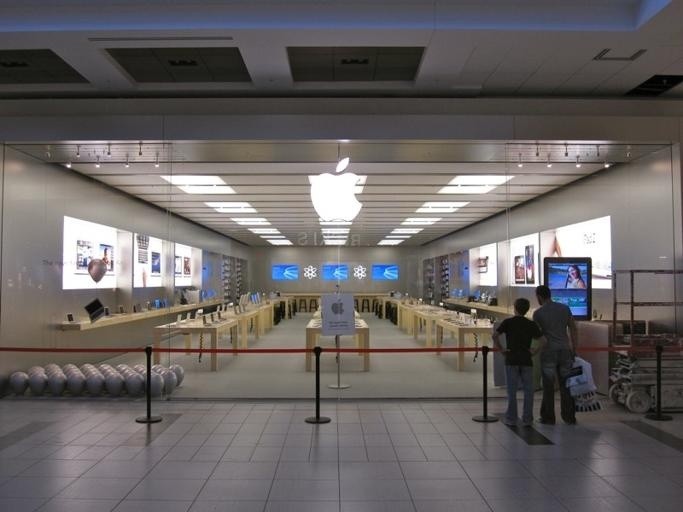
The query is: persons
[564,265,585,289]
[101,248,110,263]
[492,299,547,427]
[532,285,578,425]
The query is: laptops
[84,298,105,325]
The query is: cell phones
[120,307,124,313]
[136,234,150,263]
[76,239,94,271]
[211,314,214,322]
[276,292,280,296]
[175,256,182,277]
[99,243,114,271]
[67,314,73,321]
[104,307,109,316]
[217,312,221,320]
[203,316,207,324]
[525,245,535,284]
[515,256,525,284]
[390,291,394,297]
[148,304,152,310]
[183,257,191,276]
[133,305,136,311]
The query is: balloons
[67,375,84,397]
[106,374,123,396]
[127,374,143,395]
[29,362,169,380]
[30,374,47,395]
[170,364,184,387]
[48,374,66,395]
[89,259,107,283]
[87,375,104,396]
[9,372,29,395]
[146,374,164,397]
[164,371,177,394]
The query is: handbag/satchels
[571,358,597,397]
[574,394,602,412]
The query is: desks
[154,319,238,373]
[267,297,289,320]
[312,310,362,320]
[200,309,261,350]
[236,302,275,331]
[399,304,439,338]
[378,296,406,319]
[435,318,496,372]
[413,309,459,348]
[305,318,370,372]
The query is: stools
[373,298,380,312]
[298,299,307,312]
[362,298,370,312]
[354,299,359,312]
[309,299,318,311]
[376,302,381,316]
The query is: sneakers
[560,416,576,426]
[523,420,534,428]
[501,417,518,427]
[538,417,556,427]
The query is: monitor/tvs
[250,293,261,305]
[152,252,160,273]
[543,256,592,322]
